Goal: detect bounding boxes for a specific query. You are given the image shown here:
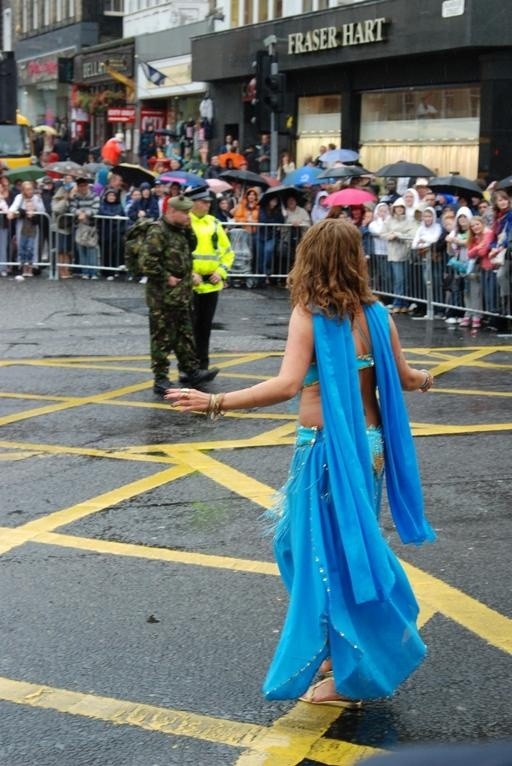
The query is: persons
[132,192,221,396]
[160,216,436,712]
[176,184,237,384]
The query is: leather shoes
[153,379,178,396]
[179,366,221,386]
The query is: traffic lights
[249,50,284,134]
[264,73,286,112]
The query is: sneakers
[81,272,149,284]
[223,278,272,290]
[385,302,512,338]
[0,265,49,277]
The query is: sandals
[297,655,362,711]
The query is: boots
[57,252,74,280]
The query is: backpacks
[122,217,164,279]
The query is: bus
[0,112,36,172]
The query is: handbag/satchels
[75,223,100,247]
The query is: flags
[138,59,168,86]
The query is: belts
[201,275,213,281]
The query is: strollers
[221,220,260,289]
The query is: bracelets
[205,391,228,421]
[419,368,432,390]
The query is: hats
[166,195,195,211]
[182,185,215,203]
